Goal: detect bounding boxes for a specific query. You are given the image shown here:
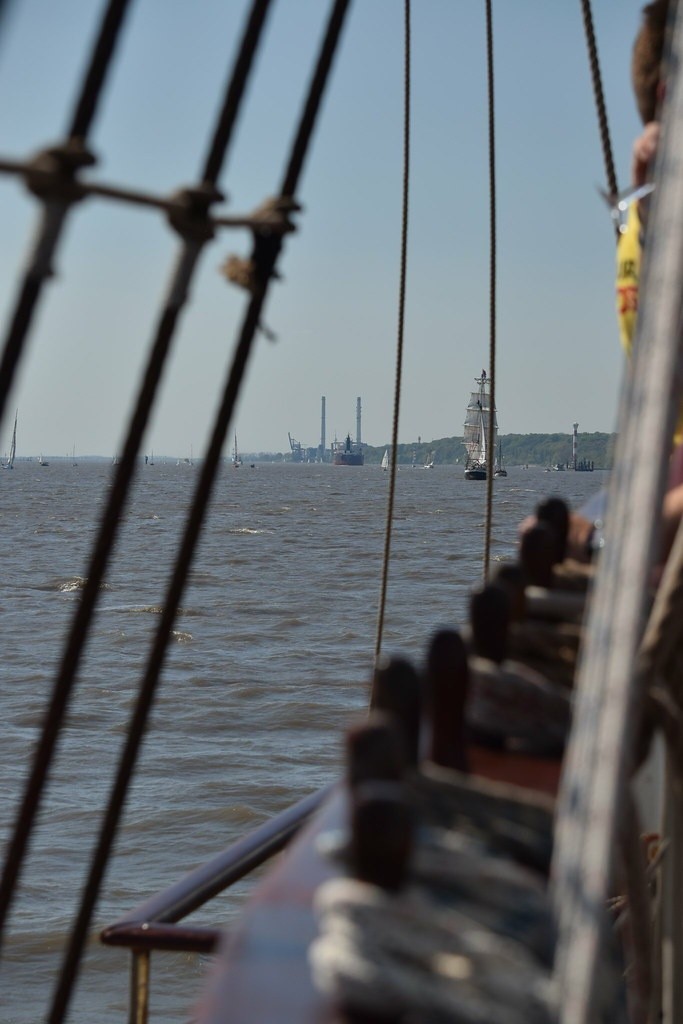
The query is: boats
[287,430,319,464]
[330,434,365,465]
[522,461,566,473]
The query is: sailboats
[494,440,507,477]
[380,450,390,471]
[0,407,18,470]
[111,451,122,465]
[462,369,499,480]
[150,448,155,465]
[70,444,77,467]
[38,450,50,466]
[411,448,436,471]
[232,428,243,469]
[175,445,194,466]
